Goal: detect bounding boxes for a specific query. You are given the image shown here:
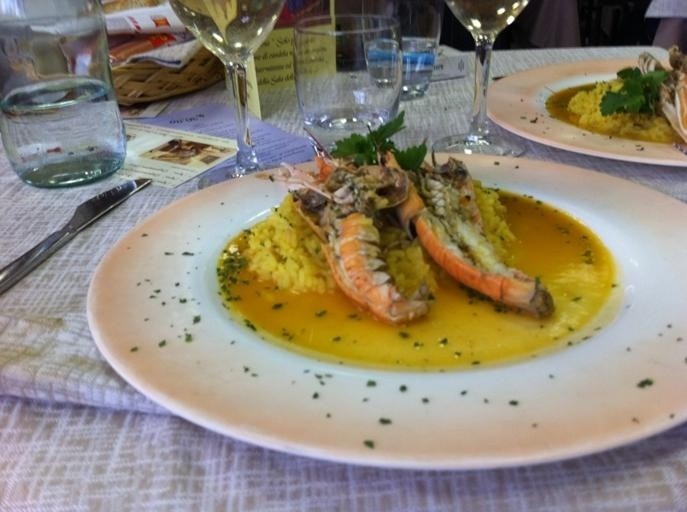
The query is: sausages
[109,34,170,63]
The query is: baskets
[110,37,227,105]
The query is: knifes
[0,178,159,298]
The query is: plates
[483,56,687,173]
[85,149,686,477]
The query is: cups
[292,1,444,153]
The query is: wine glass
[168,0,290,183]
[432,1,535,161]
